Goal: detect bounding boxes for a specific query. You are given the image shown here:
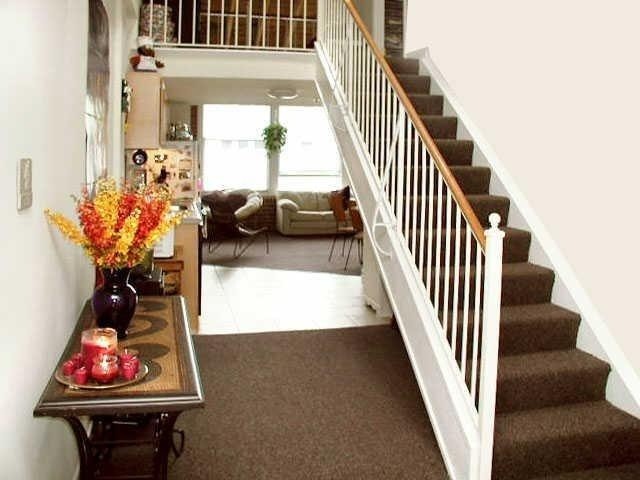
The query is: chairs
[326,194,363,272]
[203,189,273,259]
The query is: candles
[63,342,140,385]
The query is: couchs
[276,189,358,237]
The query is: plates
[54,360,149,389]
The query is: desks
[29,293,207,480]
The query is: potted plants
[263,106,288,160]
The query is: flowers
[41,173,189,271]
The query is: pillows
[278,190,305,212]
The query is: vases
[90,264,139,340]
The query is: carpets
[202,234,363,276]
[169,324,453,480]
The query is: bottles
[80,327,140,385]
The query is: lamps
[266,83,299,103]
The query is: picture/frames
[153,260,185,296]
